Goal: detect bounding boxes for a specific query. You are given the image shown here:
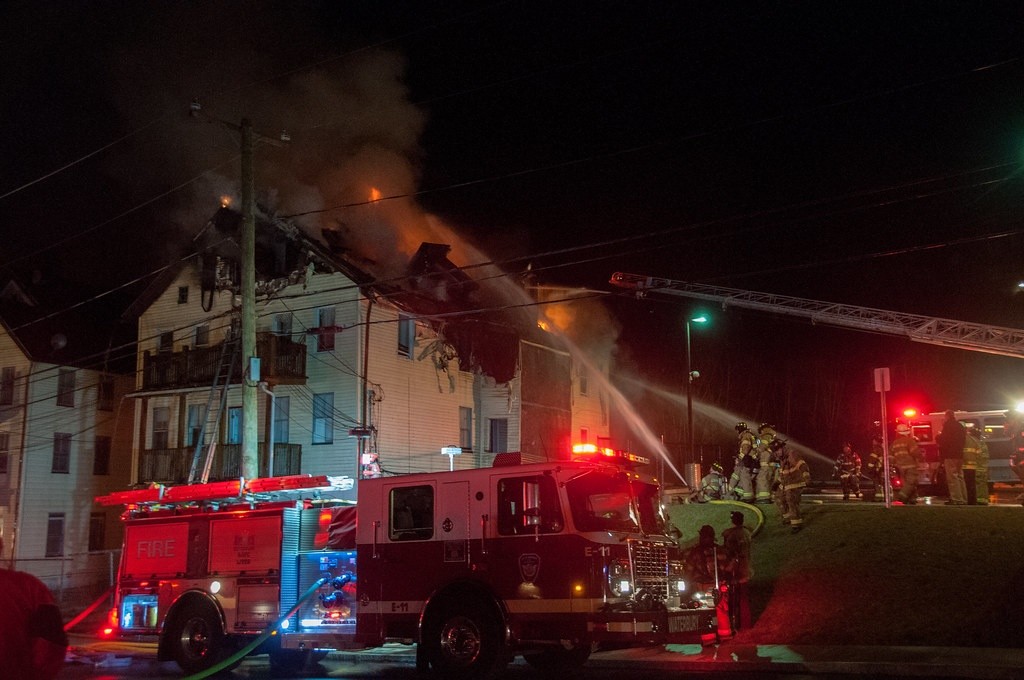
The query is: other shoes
[944,502,952,505]
[843,496,850,500]
[791,526,803,534]
[782,519,791,526]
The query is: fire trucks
[87,443,741,680]
[609,269,1024,498]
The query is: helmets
[735,422,747,434]
[895,424,909,435]
[769,439,787,453]
[730,511,744,526]
[711,462,723,473]
[666,526,682,538]
[758,424,775,434]
[697,525,718,542]
[843,442,851,448]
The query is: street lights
[441,446,462,471]
[683,315,708,488]
[348,425,372,478]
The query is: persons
[830,408,1024,507]
[683,420,812,646]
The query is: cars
[0,569,71,680]
